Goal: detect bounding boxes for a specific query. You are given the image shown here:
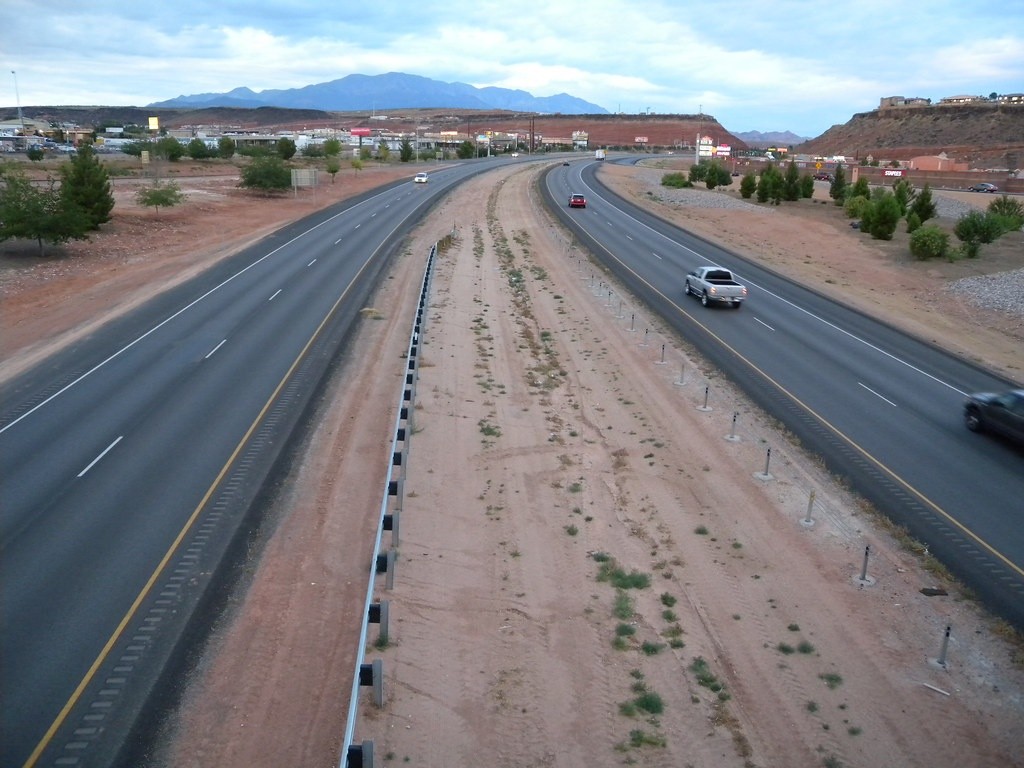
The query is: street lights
[475,130,478,158]
[416,126,419,164]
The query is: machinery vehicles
[12,71,33,136]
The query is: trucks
[596,149,607,159]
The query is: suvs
[814,172,833,181]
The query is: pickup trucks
[685,266,746,306]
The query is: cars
[568,194,588,207]
[414,172,428,183]
[965,389,1024,445]
[562,161,571,167]
[176,137,217,148]
[967,183,999,192]
[44,141,56,147]
